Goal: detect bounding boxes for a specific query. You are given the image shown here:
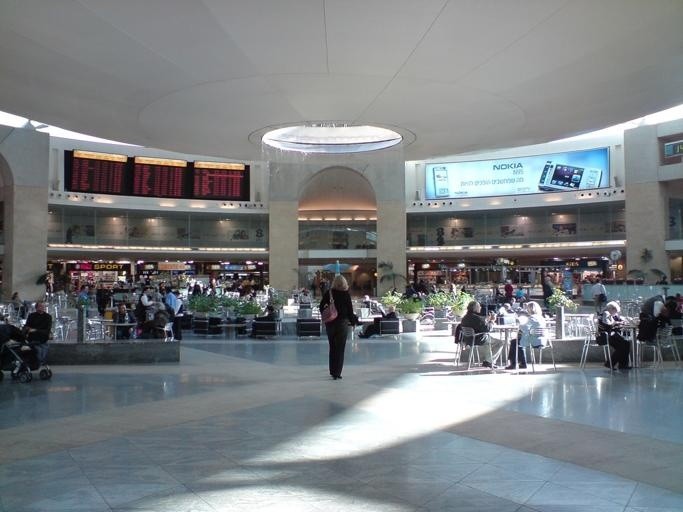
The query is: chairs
[456,325,683,371]
[0,293,174,341]
[174,311,398,339]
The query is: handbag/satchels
[599,293,607,303]
[321,288,338,324]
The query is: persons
[22,302,52,367]
[543,277,553,318]
[597,301,632,370]
[12,292,28,319]
[461,301,504,368]
[358,306,395,338]
[591,279,606,313]
[78,269,261,341]
[641,295,676,318]
[504,279,513,306]
[505,301,547,369]
[402,279,465,302]
[320,275,357,380]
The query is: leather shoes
[482,361,498,369]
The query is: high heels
[333,375,342,380]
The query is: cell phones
[434,166,448,197]
[538,161,602,191]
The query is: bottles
[125,313,129,323]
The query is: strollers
[0,322,52,382]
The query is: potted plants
[382,291,476,322]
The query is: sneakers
[519,365,527,368]
[505,366,514,369]
[604,363,632,370]
